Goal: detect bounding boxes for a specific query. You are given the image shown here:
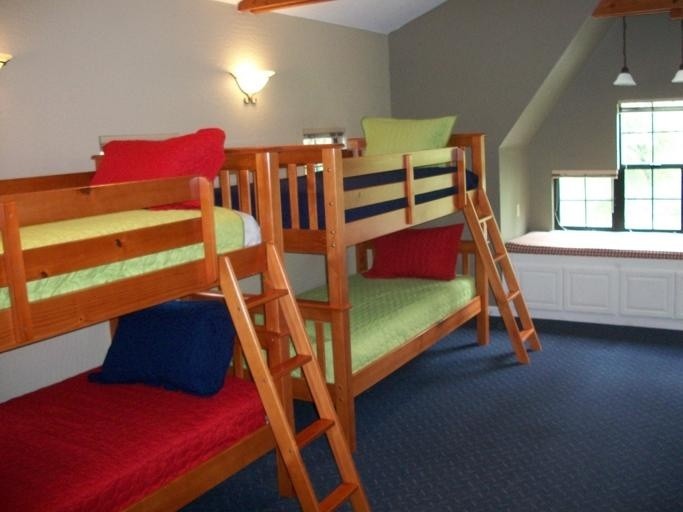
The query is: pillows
[360,222,464,280]
[90,127,227,209]
[86,300,236,398]
[360,115,458,168]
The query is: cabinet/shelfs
[504,230,683,331]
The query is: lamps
[613,16,636,86]
[670,22,683,83]
[229,63,276,105]
[0,53,13,70]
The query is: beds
[219,131,543,456]
[0,151,372,512]
